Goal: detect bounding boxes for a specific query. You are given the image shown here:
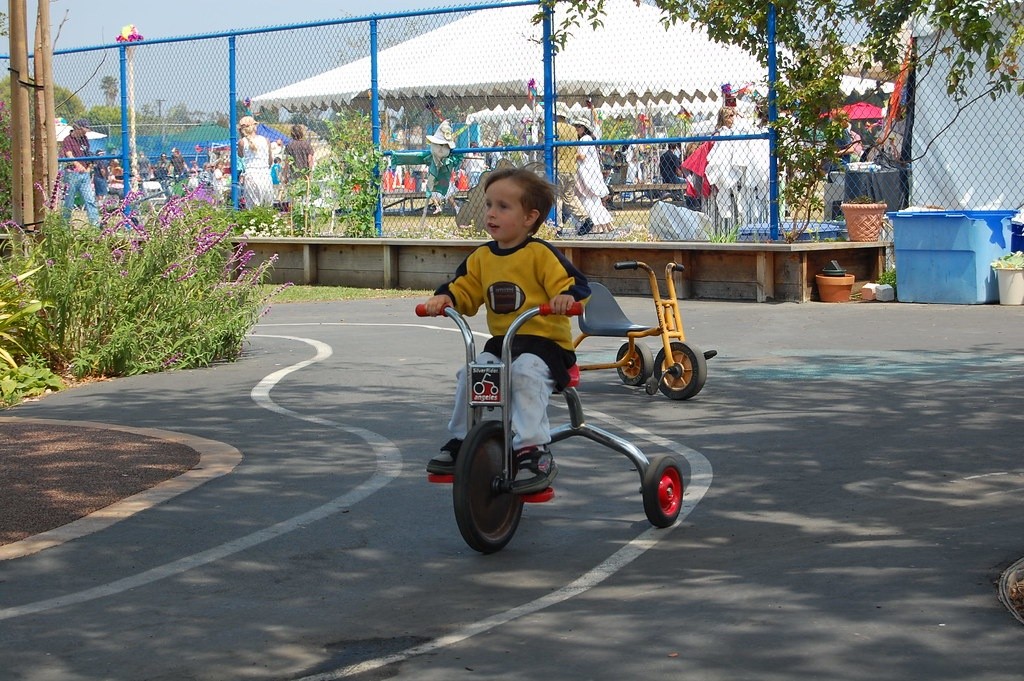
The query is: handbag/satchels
[93,175,111,195]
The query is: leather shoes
[554,229,562,238]
[577,218,594,236]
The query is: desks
[845,169,909,214]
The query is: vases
[815,260,855,302]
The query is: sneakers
[424,437,461,475]
[511,443,558,495]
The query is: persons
[238,116,272,208]
[819,111,885,172]
[63,120,124,228]
[426,168,592,494]
[601,143,699,212]
[705,106,735,134]
[138,147,226,207]
[552,102,614,237]
[432,139,505,214]
[271,125,314,211]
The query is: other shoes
[432,208,442,214]
[455,207,461,215]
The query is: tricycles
[574,259,718,400]
[413,301,685,555]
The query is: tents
[245,0,897,171]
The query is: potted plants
[841,194,887,242]
[990,251,1024,305]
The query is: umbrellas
[821,102,885,128]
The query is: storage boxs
[886,210,1022,305]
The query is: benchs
[609,184,688,209]
[382,191,468,213]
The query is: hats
[550,102,569,119]
[71,119,92,132]
[573,118,594,132]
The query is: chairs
[577,282,651,337]
[138,181,168,215]
